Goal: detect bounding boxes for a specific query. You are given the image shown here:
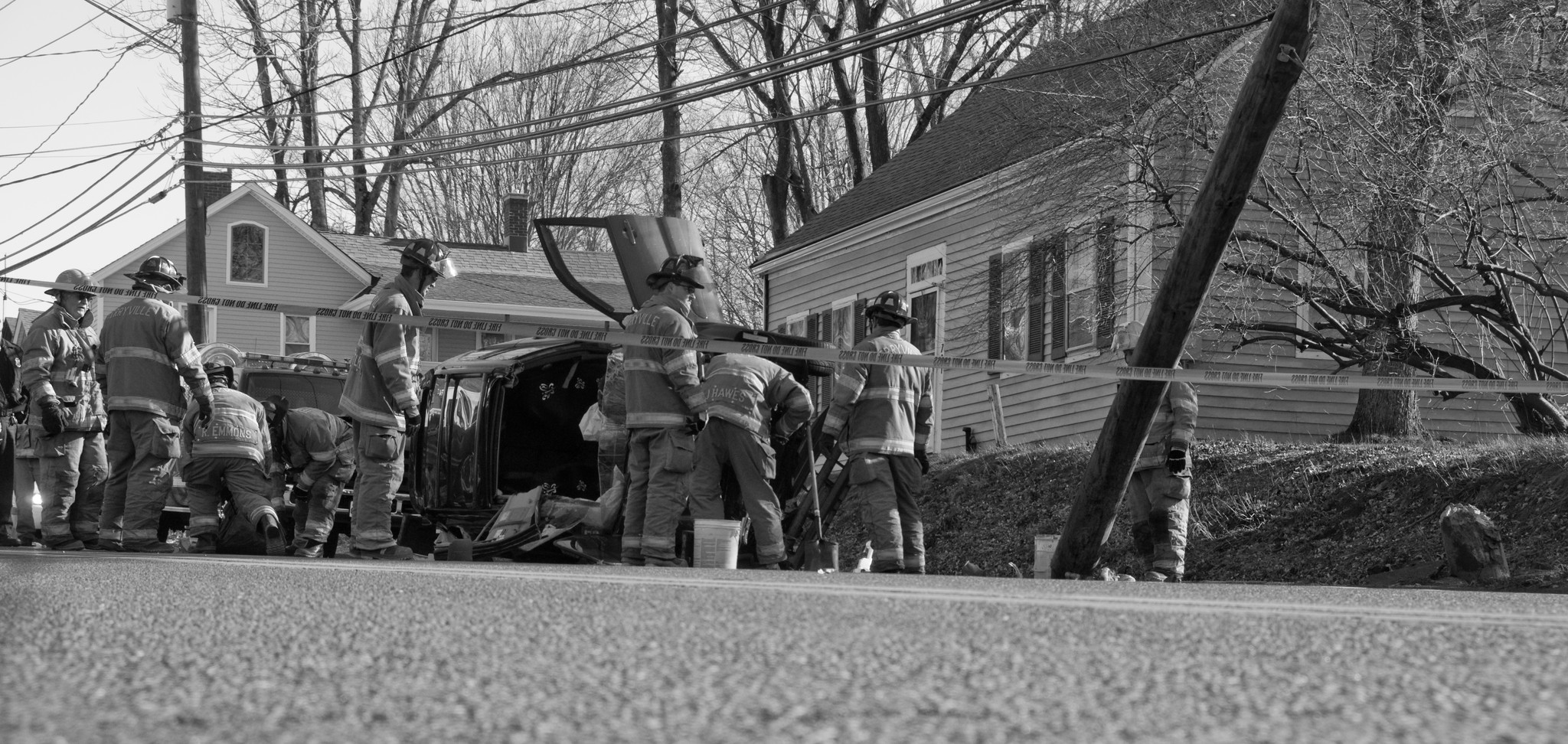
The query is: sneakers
[351,539,412,559]
[123,538,175,553]
[98,538,127,553]
[645,555,688,568]
[1,534,20,546]
[264,514,285,554]
[186,534,220,553]
[81,537,102,549]
[855,557,902,573]
[51,538,83,550]
[295,540,325,557]
[621,549,646,565]
[286,543,300,556]
[18,535,32,545]
[1142,571,1182,583]
[903,561,926,574]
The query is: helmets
[861,290,918,324]
[1113,321,1144,354]
[198,362,235,388]
[646,254,713,290]
[43,270,97,299]
[124,256,191,295]
[259,394,290,436]
[402,238,460,280]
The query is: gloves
[292,484,311,502]
[406,405,421,437]
[915,450,930,476]
[41,400,69,435]
[199,402,215,430]
[1167,451,1186,470]
[685,409,709,437]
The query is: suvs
[409,211,846,577]
[158,346,415,569]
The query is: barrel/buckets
[1033,534,1062,580]
[693,518,742,569]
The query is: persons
[688,352,816,569]
[21,268,115,551]
[260,394,356,557]
[14,408,42,545]
[337,238,460,557]
[93,255,216,553]
[1,336,24,546]
[816,289,935,574]
[620,254,711,568]
[178,373,290,555]
[1110,321,1198,582]
